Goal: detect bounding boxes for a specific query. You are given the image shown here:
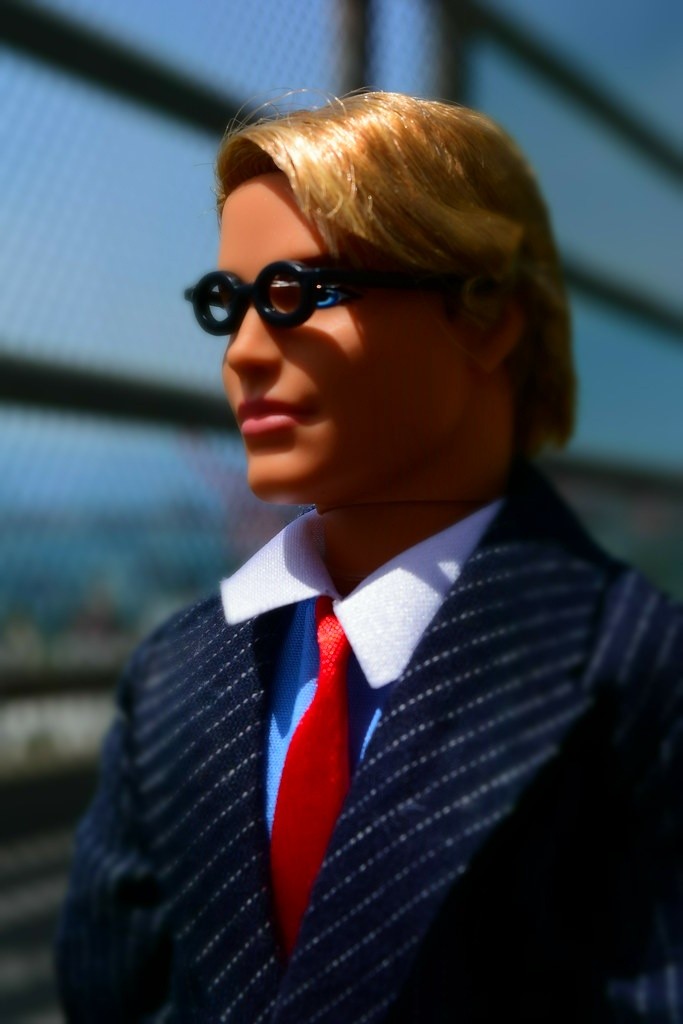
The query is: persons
[55,91,683,1024]
